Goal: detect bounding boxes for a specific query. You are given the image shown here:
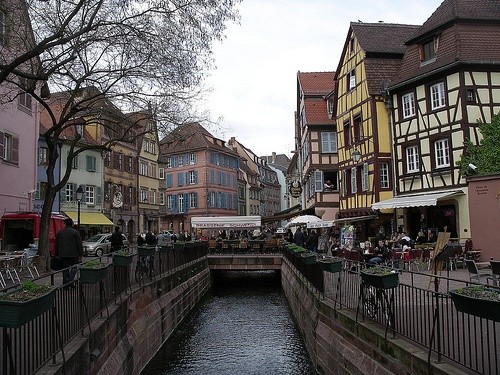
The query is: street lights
[76,185,84,236]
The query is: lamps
[351,148,363,166]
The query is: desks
[411,247,434,263]
[476,262,498,286]
[396,251,410,270]
[466,250,481,263]
[363,253,373,263]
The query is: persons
[323,180,334,189]
[54,218,82,290]
[137,227,439,272]
[110,225,124,251]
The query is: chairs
[335,243,500,287]
[0,250,40,289]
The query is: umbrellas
[286,215,322,231]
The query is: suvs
[161,230,180,240]
[82,234,129,257]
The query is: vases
[138,247,157,256]
[248,240,266,245]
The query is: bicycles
[135,255,156,282]
[360,284,394,328]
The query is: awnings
[371,190,466,211]
[307,216,373,228]
[62,211,115,225]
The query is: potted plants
[185,240,208,249]
[174,240,185,251]
[282,240,319,265]
[318,256,345,273]
[159,242,173,254]
[222,239,240,244]
[112,248,135,268]
[448,285,500,324]
[359,267,399,289]
[0,278,59,329]
[75,257,112,284]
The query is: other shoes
[70,284,76,288]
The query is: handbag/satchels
[50,258,62,270]
